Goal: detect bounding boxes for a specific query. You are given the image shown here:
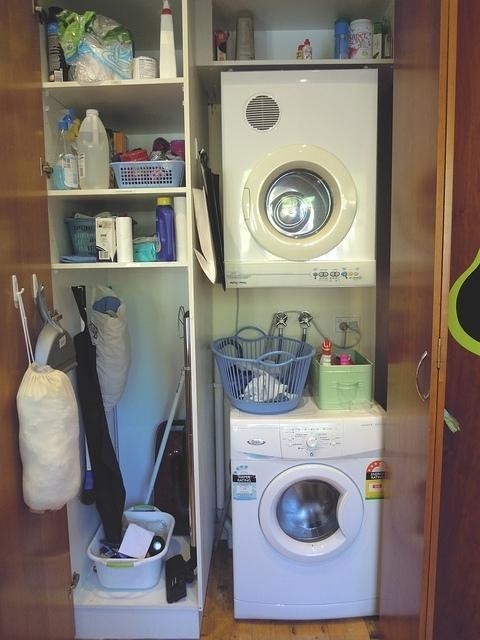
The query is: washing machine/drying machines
[221,68,377,290]
[380,382,430,619]
[229,382,385,622]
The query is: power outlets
[335,314,360,333]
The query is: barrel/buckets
[75,109,110,189]
[155,196,176,262]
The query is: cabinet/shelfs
[0,0,457,640]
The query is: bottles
[51,122,78,190]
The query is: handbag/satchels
[17,362,81,512]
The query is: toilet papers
[174,196,188,262]
[115,217,134,262]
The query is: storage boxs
[86,503,176,590]
[308,349,373,410]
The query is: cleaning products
[69,118,81,142]
[159,0,177,79]
[51,113,79,191]
[67,107,76,119]
[319,338,331,367]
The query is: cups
[334,19,351,60]
[134,241,156,262]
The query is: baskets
[213,326,315,412]
[65,216,96,254]
[113,159,184,188]
